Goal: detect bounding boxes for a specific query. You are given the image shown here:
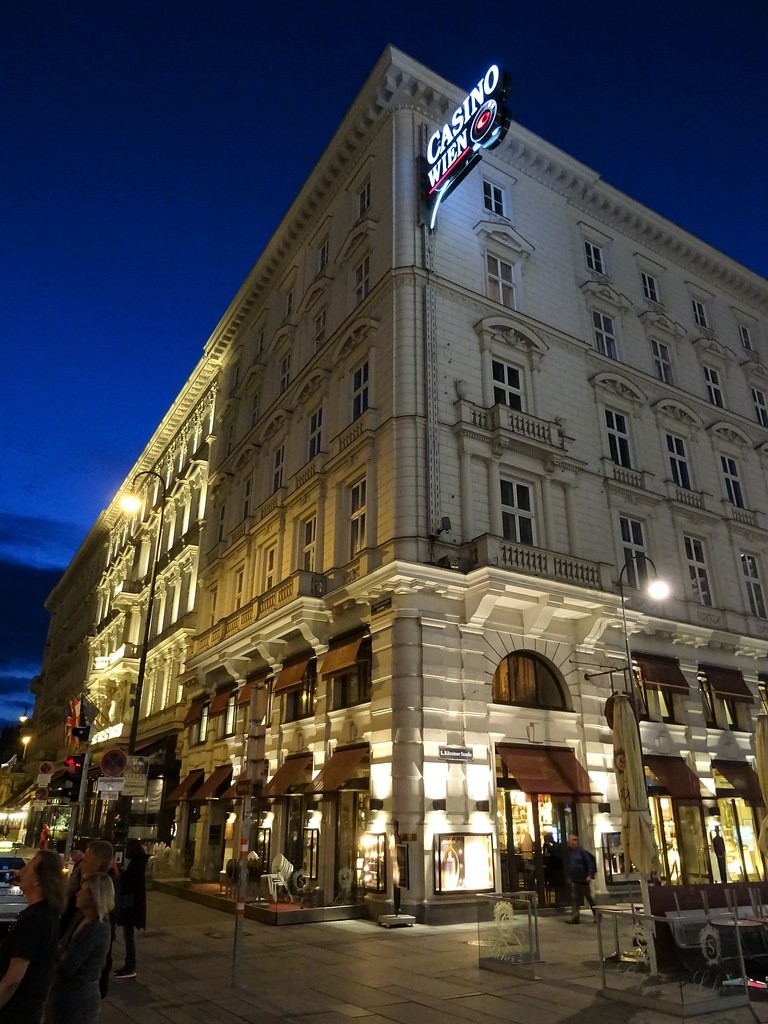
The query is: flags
[64,692,100,746]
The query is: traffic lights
[71,726,92,741]
[62,752,86,803]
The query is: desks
[680,918,768,995]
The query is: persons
[38,822,52,848]
[546,835,560,903]
[563,836,603,923]
[0,837,148,1024]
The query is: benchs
[665,904,768,977]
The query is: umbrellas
[612,689,663,972]
[756,713,768,858]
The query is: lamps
[432,517,451,540]
[708,806,721,817]
[434,799,446,812]
[306,800,319,814]
[598,802,611,814]
[475,801,490,812]
[369,799,384,812]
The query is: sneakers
[113,968,137,978]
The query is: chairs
[258,853,295,905]
[149,841,170,874]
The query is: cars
[0,833,28,921]
[57,853,75,873]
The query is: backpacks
[580,847,598,880]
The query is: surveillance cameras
[442,516,451,530]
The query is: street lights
[116,468,171,756]
[617,552,671,712]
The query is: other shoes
[565,917,580,924]
[593,915,602,924]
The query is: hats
[711,826,721,831]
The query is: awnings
[711,760,766,807]
[644,758,718,806]
[495,746,604,804]
[236,672,266,708]
[251,756,311,799]
[320,629,368,681]
[219,768,262,801]
[183,697,204,727]
[209,688,234,719]
[272,657,308,698]
[303,747,370,795]
[188,766,228,804]
[164,771,201,806]
